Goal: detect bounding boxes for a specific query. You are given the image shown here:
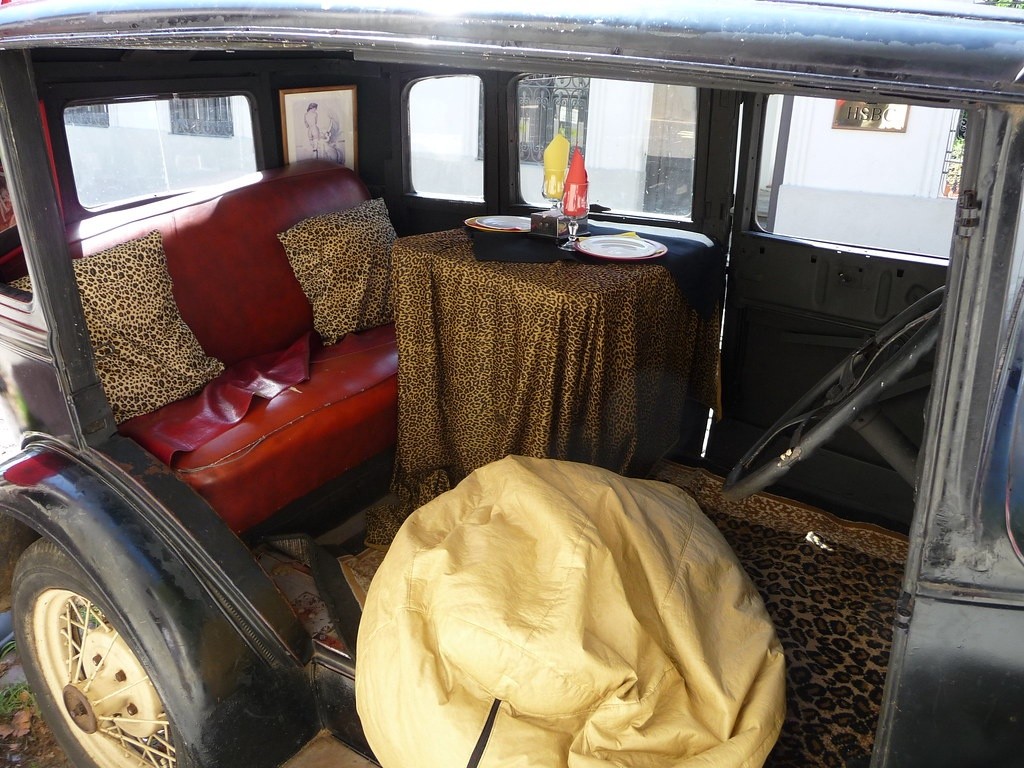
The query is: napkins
[543,132,570,201]
[565,146,587,215]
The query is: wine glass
[541,167,569,211]
[556,180,590,251]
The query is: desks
[388,224,712,521]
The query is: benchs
[0,160,396,533]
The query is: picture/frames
[279,87,357,172]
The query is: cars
[0,0,1024,768]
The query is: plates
[464,216,532,232]
[578,236,656,257]
[574,235,668,261]
[476,216,530,230]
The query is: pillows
[8,228,226,425]
[275,196,398,346]
